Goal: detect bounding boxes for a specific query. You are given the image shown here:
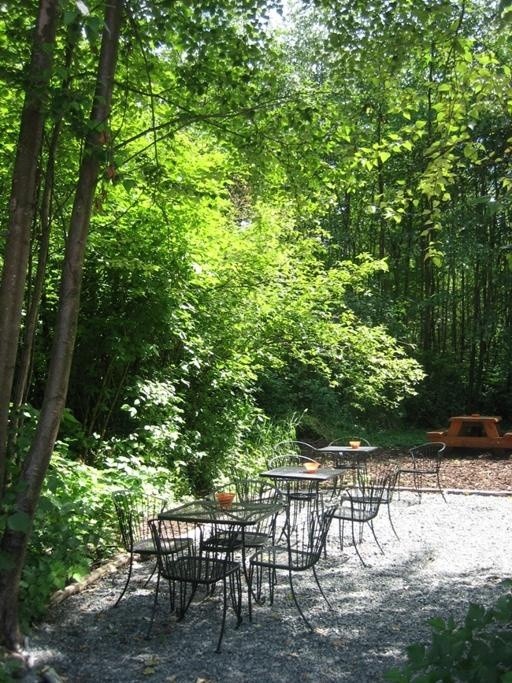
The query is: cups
[305,463,320,473]
[217,493,236,506]
[350,442,360,448]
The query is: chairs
[328,436,372,475]
[143,518,242,651]
[248,504,337,631]
[111,491,197,609]
[273,441,324,469]
[396,441,448,506]
[269,458,322,530]
[339,466,401,546]
[212,480,282,584]
[321,477,386,565]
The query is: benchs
[427,427,447,446]
[501,428,512,450]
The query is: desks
[157,501,282,606]
[448,416,502,457]
[315,446,378,494]
[260,466,346,546]
